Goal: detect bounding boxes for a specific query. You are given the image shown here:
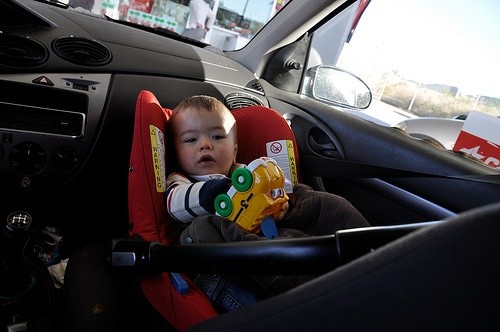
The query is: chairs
[62,197,499,332]
[110,90,459,332]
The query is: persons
[161,94,378,296]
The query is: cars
[215,157,290,232]
